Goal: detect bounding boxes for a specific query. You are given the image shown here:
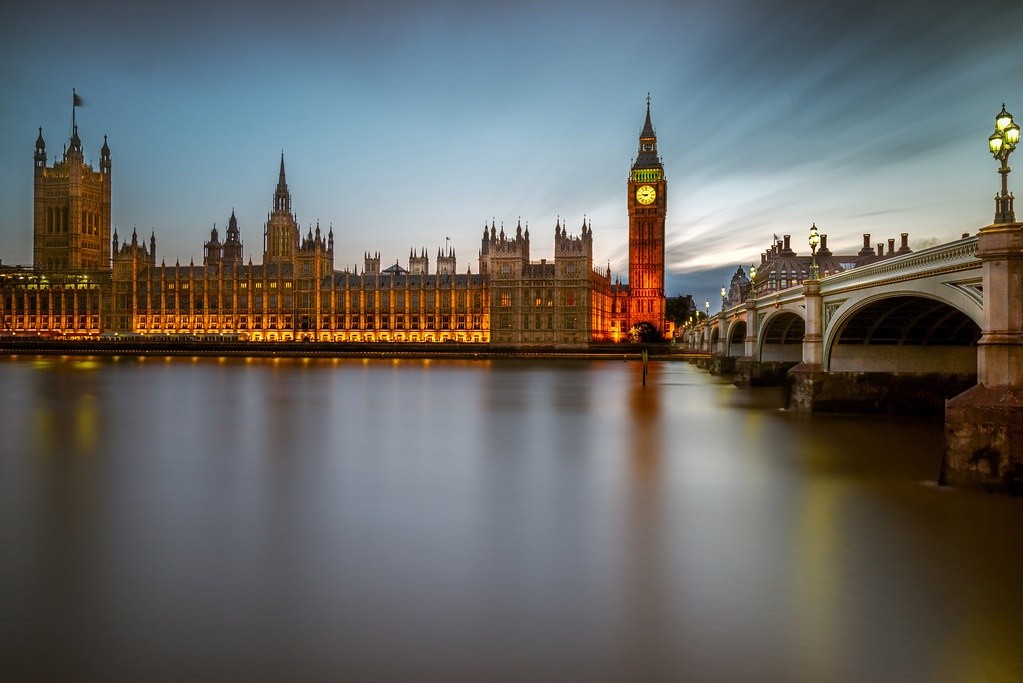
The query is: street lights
[988,102,1020,224]
[682,316,693,333]
[809,221,820,280]
[720,285,726,312]
[750,263,756,300]
[706,300,709,318]
[696,309,700,325]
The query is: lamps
[989,102,1019,159]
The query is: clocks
[636,185,656,205]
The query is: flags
[73,92,83,106]
